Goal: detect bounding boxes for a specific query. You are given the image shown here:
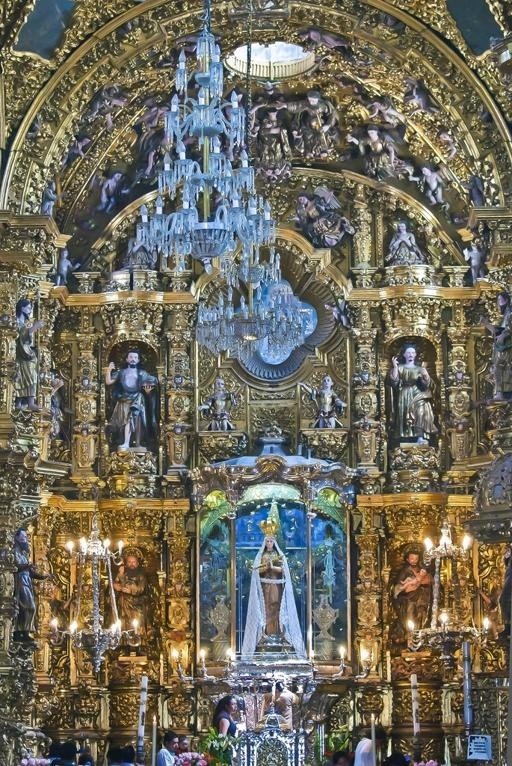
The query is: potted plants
[326,723,355,766]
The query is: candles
[151,715,157,766]
[370,712,377,766]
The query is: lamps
[170,627,377,731]
[185,0,318,351]
[130,2,275,274]
[404,514,493,674]
[47,496,141,676]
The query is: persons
[297,375,346,429]
[157,732,205,765]
[487,293,511,349]
[213,697,237,765]
[199,378,237,430]
[333,727,438,765]
[44,737,135,765]
[390,344,436,444]
[8,529,47,632]
[260,671,299,727]
[115,553,146,626]
[15,300,43,410]
[324,302,351,328]
[392,550,430,632]
[239,531,307,653]
[44,32,488,285]
[106,351,156,447]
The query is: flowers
[22,754,60,766]
[411,756,440,766]
[172,750,211,766]
